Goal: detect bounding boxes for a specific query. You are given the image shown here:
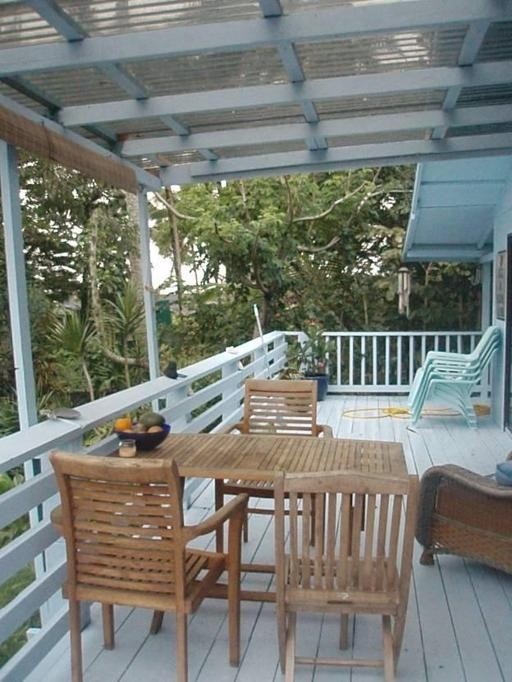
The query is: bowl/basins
[112,422,170,451]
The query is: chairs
[272,466,420,681]
[51,448,251,682]
[212,378,335,556]
[404,325,503,432]
[414,449,512,576]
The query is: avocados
[140,413,165,427]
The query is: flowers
[297,317,337,376]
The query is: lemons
[114,418,131,431]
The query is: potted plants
[279,342,313,414]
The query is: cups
[118,439,136,458]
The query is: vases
[305,373,330,401]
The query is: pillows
[494,460,512,485]
